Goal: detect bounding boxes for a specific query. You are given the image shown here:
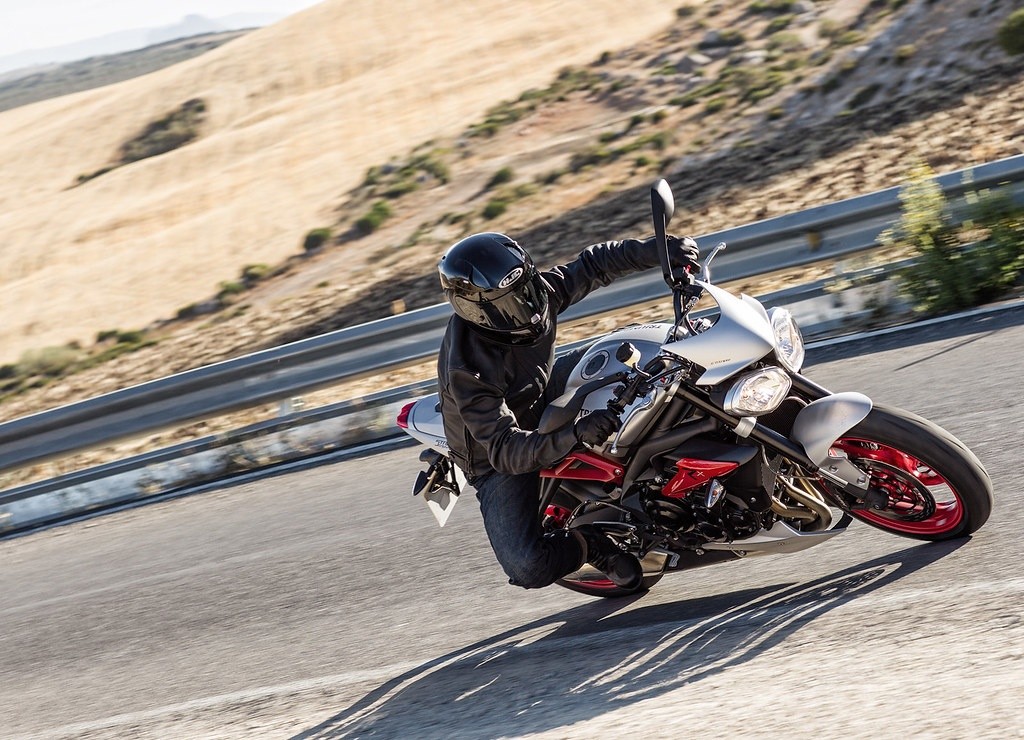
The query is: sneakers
[581,531,642,592]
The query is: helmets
[437,232,554,347]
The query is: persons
[434,232,699,592]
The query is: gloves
[575,409,622,449]
[645,235,702,273]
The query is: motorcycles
[397,178,996,600]
